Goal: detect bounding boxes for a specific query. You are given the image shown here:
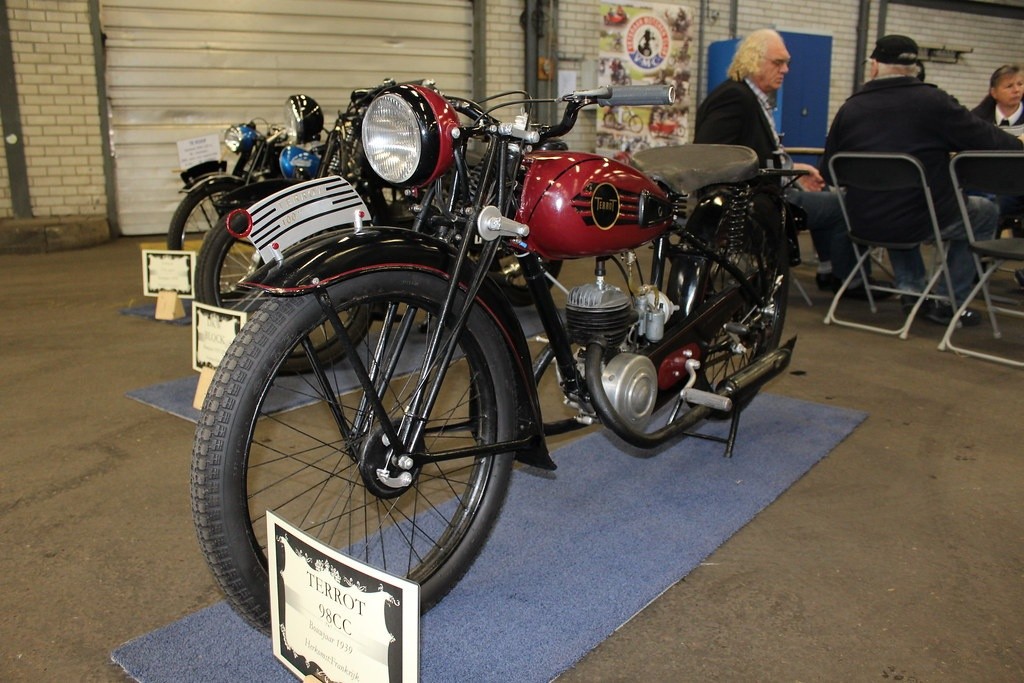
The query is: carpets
[108,382,869,683]
[117,290,567,428]
[119,294,196,330]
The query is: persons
[698,28,891,301]
[959,62,1023,295]
[820,33,1023,327]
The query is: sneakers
[816,272,833,288]
[830,276,894,298]
[896,281,934,313]
[922,297,983,326]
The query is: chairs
[937,149,1023,367]
[822,150,962,341]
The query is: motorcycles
[168,116,480,300]
[191,77,796,637]
[193,87,569,374]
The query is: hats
[860,34,919,65]
